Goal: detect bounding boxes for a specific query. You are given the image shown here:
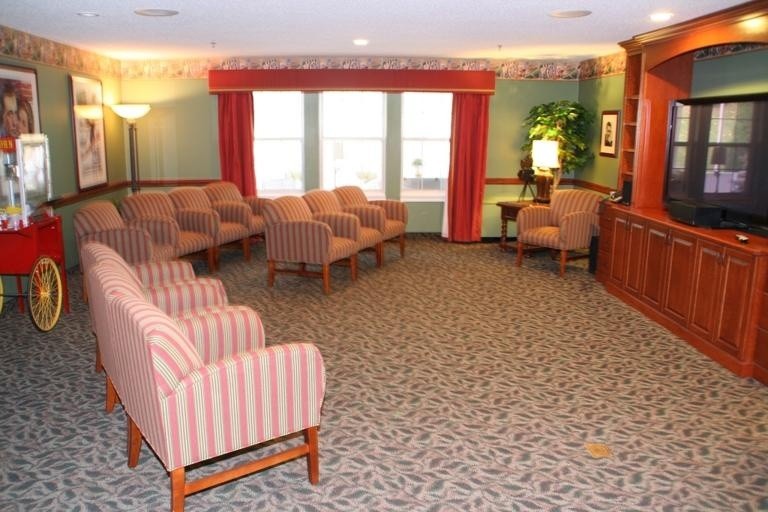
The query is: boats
[622,180,632,206]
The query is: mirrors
[15,139,53,228]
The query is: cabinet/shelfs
[617,49,692,210]
[598,194,768,386]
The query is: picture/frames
[599,110,620,157]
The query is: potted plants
[599,110,620,157]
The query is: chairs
[72,181,271,275]
[262,186,407,296]
[81,242,326,511]
[515,190,603,276]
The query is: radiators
[736,234,748,241]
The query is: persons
[16,100,34,136]
[604,121,612,147]
[0,87,18,137]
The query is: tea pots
[15,139,53,228]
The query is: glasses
[111,104,151,191]
[531,139,560,204]
[0,63,48,202]
[69,77,108,191]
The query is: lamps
[69,77,108,191]
[531,139,560,204]
[0,63,48,202]
[515,190,603,276]
[111,104,151,191]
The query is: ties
[661,92,768,236]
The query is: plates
[598,194,768,386]
[617,49,692,210]
